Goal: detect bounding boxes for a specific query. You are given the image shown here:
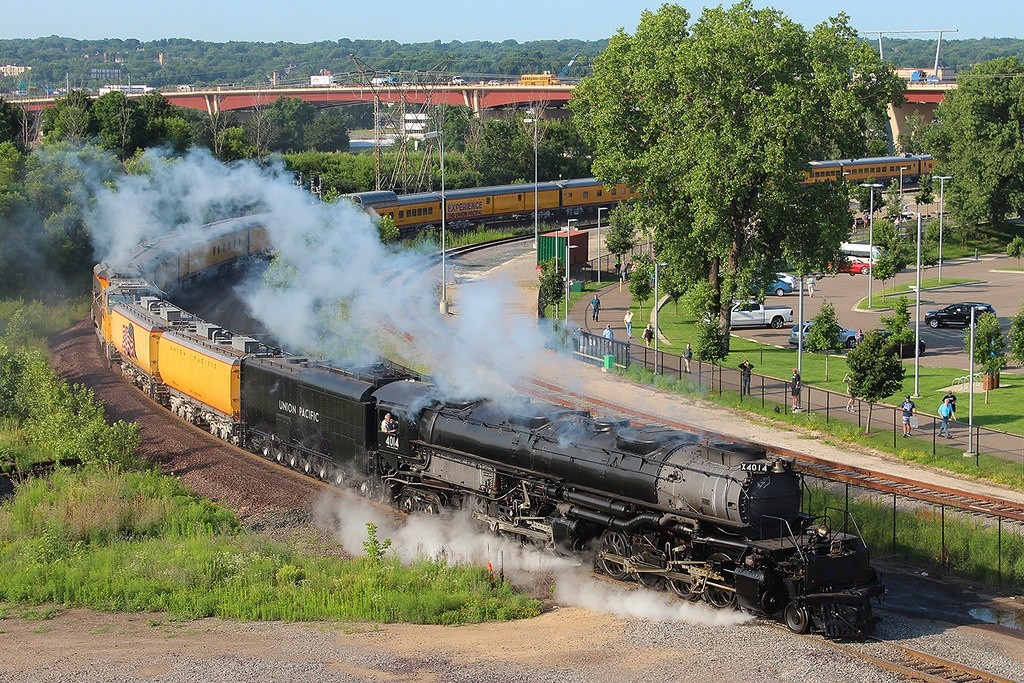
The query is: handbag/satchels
[910,414,918,428]
[952,402,955,412]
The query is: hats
[906,395,910,399]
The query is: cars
[744,271,797,297]
[488,80,503,87]
[835,255,874,274]
[874,329,927,359]
[788,321,857,352]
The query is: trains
[90,154,936,642]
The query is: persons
[682,343,692,374]
[381,413,398,433]
[790,368,800,411]
[591,294,600,322]
[624,310,634,339]
[942,390,957,428]
[938,399,956,439]
[620,261,628,285]
[900,394,916,437]
[738,360,754,396]
[642,324,654,350]
[843,373,856,413]
[602,324,615,355]
[806,275,816,297]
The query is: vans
[838,240,888,264]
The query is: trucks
[311,75,340,88]
[370,75,400,86]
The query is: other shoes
[908,433,913,436]
[846,407,851,413]
[790,405,797,410]
[938,434,944,437]
[902,434,906,437]
[947,434,953,439]
[851,408,854,412]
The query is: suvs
[924,301,996,329]
[449,75,467,86]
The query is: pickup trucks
[704,298,794,332]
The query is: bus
[520,73,561,86]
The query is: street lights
[968,306,988,454]
[901,211,922,393]
[654,263,668,374]
[900,167,908,196]
[597,207,608,283]
[932,176,953,282]
[526,118,538,250]
[859,184,884,309]
[565,219,578,301]
[423,129,448,316]
[565,246,578,322]
